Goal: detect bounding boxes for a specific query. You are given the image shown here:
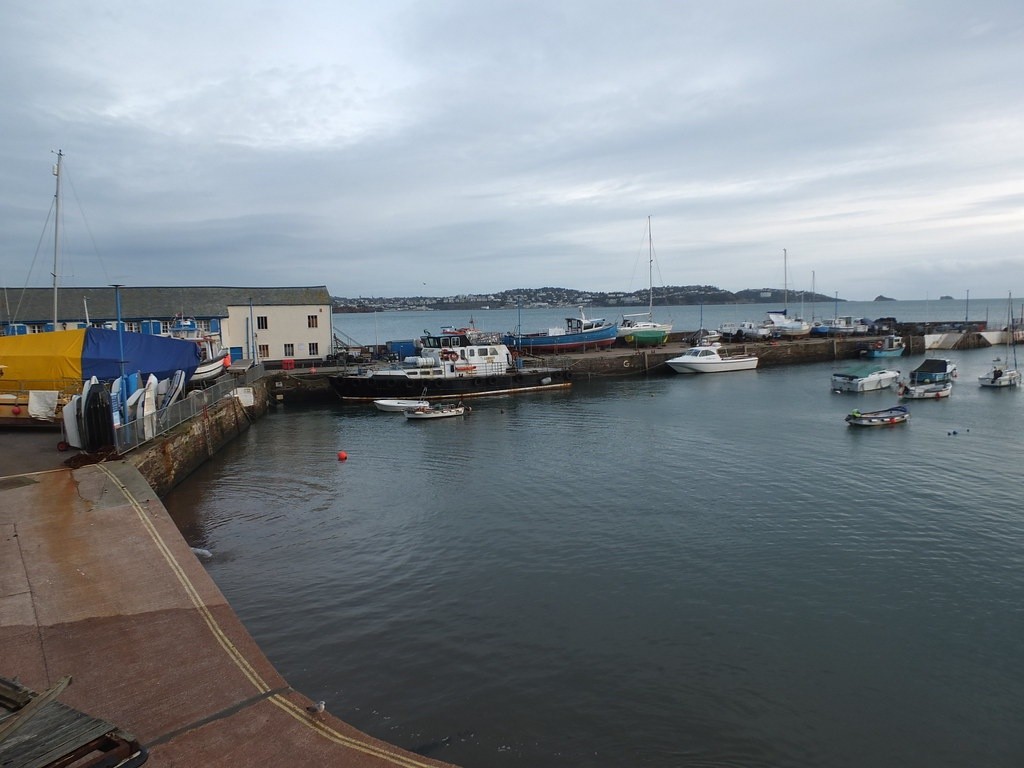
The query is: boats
[897,382,954,401]
[666,345,760,375]
[844,403,911,426]
[399,405,469,418]
[830,367,899,396]
[369,398,430,411]
[908,355,958,385]
[860,342,904,359]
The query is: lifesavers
[450,353,459,362]
[441,349,449,359]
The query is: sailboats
[976,289,1022,390]
[407,211,869,370]
